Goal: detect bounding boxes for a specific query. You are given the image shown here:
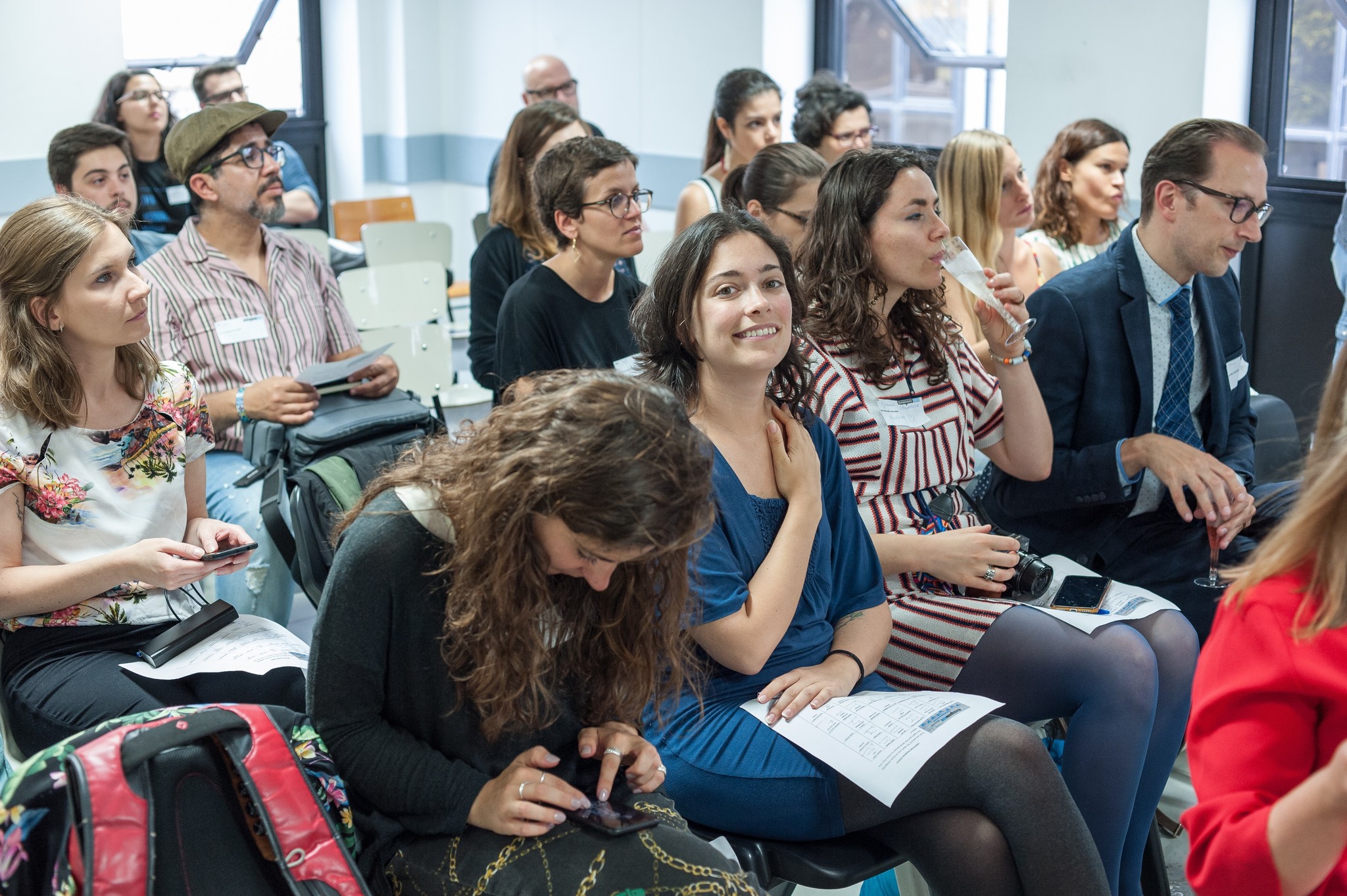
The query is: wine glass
[1193,477,1235,588]
[935,235,1037,347]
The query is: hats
[164,102,288,188]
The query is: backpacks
[0,702,374,896]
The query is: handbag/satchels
[240,382,446,473]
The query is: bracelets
[235,387,254,424]
[987,335,1033,365]
[823,649,864,684]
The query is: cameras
[977,528,1052,599]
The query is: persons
[136,103,401,632]
[765,151,1200,896]
[467,54,1132,513]
[1180,344,1347,896]
[1332,190,1347,369]
[51,66,460,385]
[626,213,1114,896]
[979,118,1303,644]
[0,197,307,757]
[307,368,769,896]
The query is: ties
[1155,287,1208,506]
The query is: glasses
[1169,178,1273,228]
[824,123,879,149]
[525,79,577,101]
[580,189,653,219]
[114,90,170,108]
[202,86,245,103]
[195,143,286,176]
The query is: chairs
[0,195,1299,896]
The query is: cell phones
[1050,575,1113,613]
[564,793,660,837]
[177,541,259,565]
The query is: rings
[984,564,996,581]
[656,764,667,777]
[519,781,532,800]
[603,746,624,761]
[539,771,546,784]
